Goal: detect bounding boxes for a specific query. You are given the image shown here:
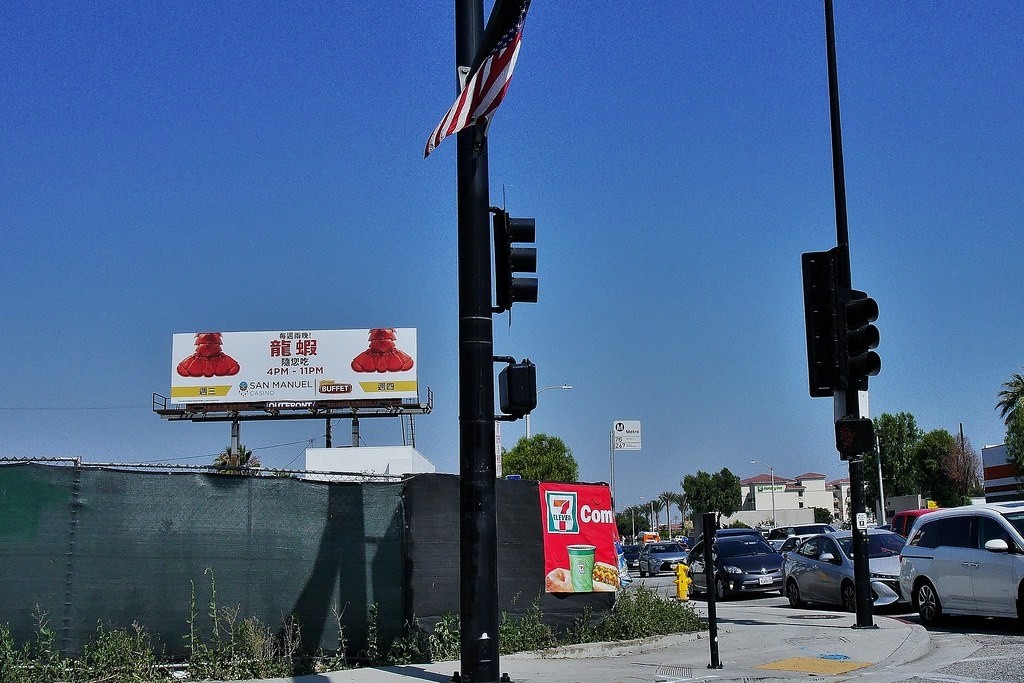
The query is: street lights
[525,385,574,439]
[750,460,777,529]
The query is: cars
[674,534,691,553]
[775,533,821,555]
[620,545,643,571]
[694,528,777,553]
[780,528,913,613]
[763,522,844,554]
[680,534,785,602]
[880,507,947,555]
[898,500,1024,631]
[637,541,689,578]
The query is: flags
[425,0,532,159]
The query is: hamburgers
[591,561,620,591]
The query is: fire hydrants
[672,562,693,602]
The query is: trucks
[635,530,661,549]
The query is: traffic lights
[833,297,882,391]
[498,357,537,414]
[493,208,540,308]
[835,415,875,455]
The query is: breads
[545,567,573,592]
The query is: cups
[565,544,597,592]
